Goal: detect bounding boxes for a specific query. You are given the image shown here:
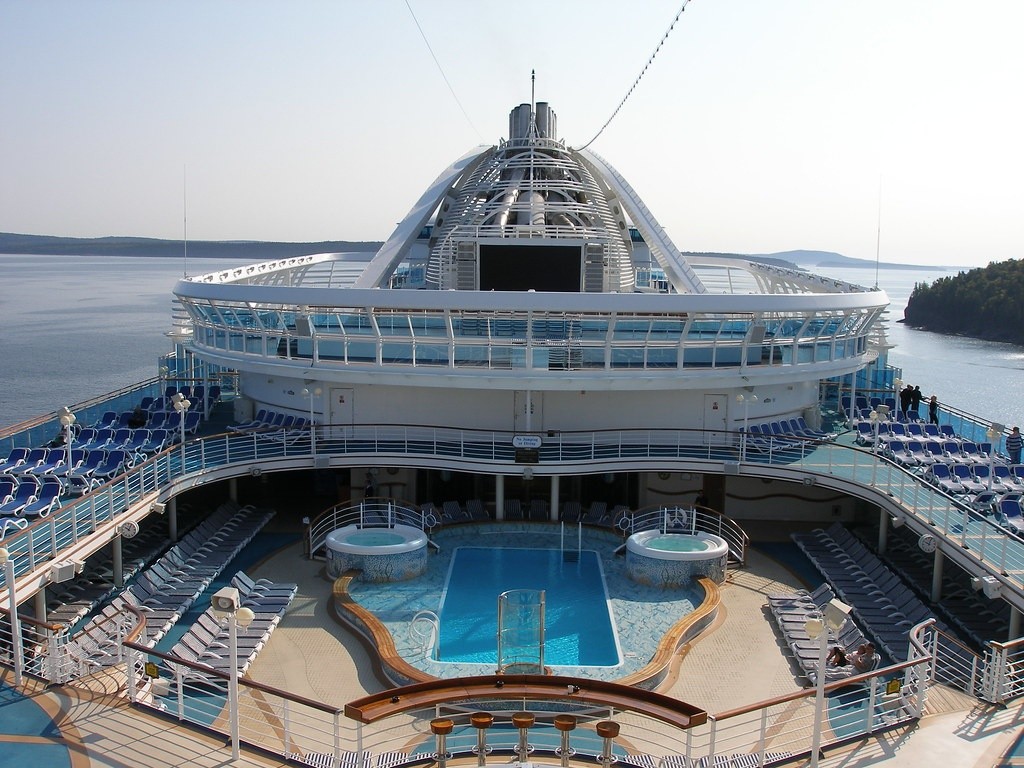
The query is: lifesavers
[666,506,688,528]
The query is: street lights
[736,394,758,464]
[60,413,76,478]
[172,393,191,474]
[872,404,889,488]
[985,430,1002,496]
[208,587,254,759]
[800,599,853,768]
[302,387,322,455]
[159,366,169,409]
[893,378,904,420]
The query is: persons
[813,642,877,678]
[826,643,865,665]
[59,426,73,440]
[900,384,926,420]
[929,396,940,427]
[1005,427,1024,464]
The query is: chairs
[738,395,1024,693]
[0,385,317,682]
[400,507,433,528]
[364,512,382,523]
[467,500,491,521]
[381,508,409,524]
[559,500,692,533]
[528,500,549,520]
[504,499,523,521]
[420,502,458,524]
[443,500,472,523]
